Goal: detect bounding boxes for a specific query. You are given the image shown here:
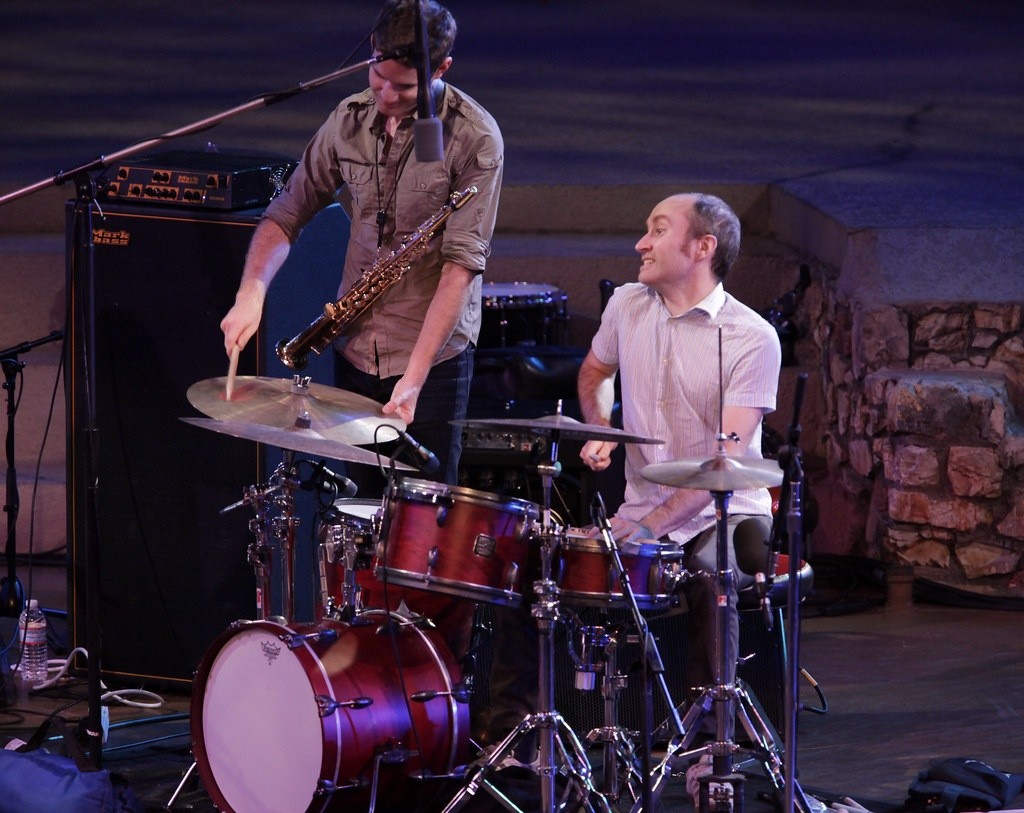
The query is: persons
[219,0,505,497]
[577,192,782,809]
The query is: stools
[737,553,815,782]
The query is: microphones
[412,13,442,163]
[398,430,441,476]
[754,569,775,631]
[298,458,359,498]
[1,326,63,362]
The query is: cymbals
[175,415,423,481]
[455,411,664,456]
[193,373,408,446]
[637,455,785,491]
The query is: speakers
[63,200,351,695]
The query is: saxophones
[273,186,479,372]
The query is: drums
[482,278,572,357]
[323,496,462,632]
[376,477,541,612]
[191,607,470,812]
[564,532,683,614]
